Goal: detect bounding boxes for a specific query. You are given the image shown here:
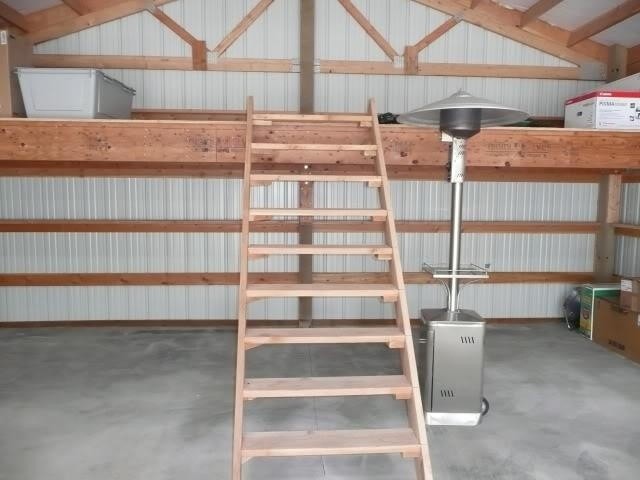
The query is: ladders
[231,97,433,480]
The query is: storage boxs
[579,282,620,340]
[13,63,136,120]
[564,91,640,130]
[620,277,640,312]
[593,296,640,364]
[0,29,34,117]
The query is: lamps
[395,91,528,423]
